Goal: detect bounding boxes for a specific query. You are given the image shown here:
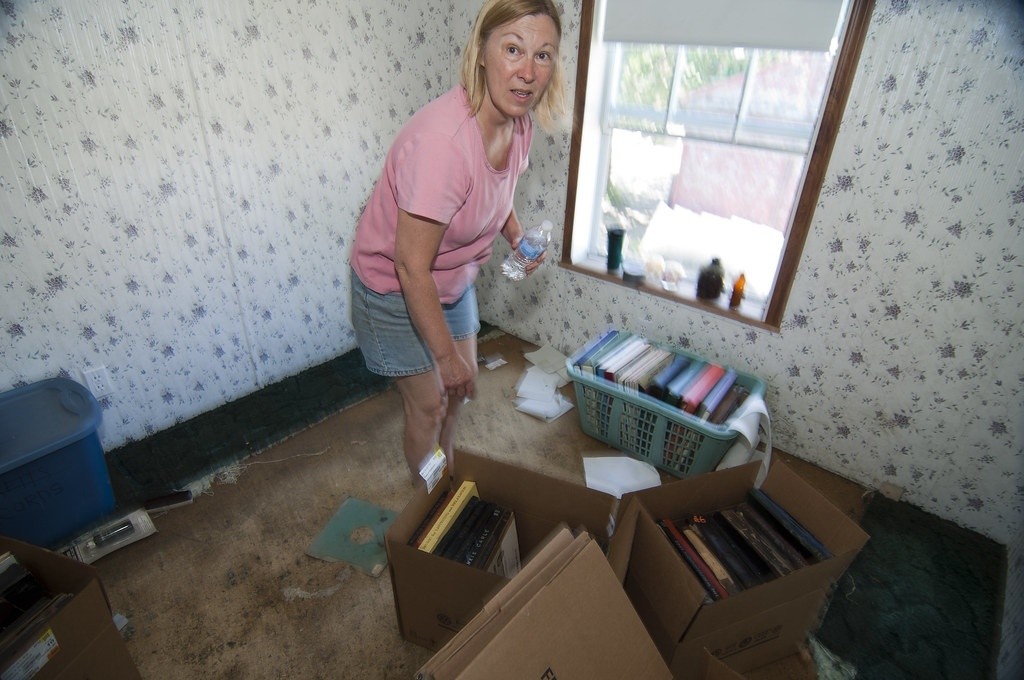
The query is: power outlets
[82,365,115,400]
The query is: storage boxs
[0,536,140,680]
[0,377,116,548]
[565,330,768,480]
[614,453,870,680]
[384,446,639,652]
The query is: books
[573,329,832,604]
[0,549,75,671]
[407,480,525,583]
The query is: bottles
[695,260,722,299]
[501,220,553,281]
[730,274,746,309]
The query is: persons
[351,0,569,490]
